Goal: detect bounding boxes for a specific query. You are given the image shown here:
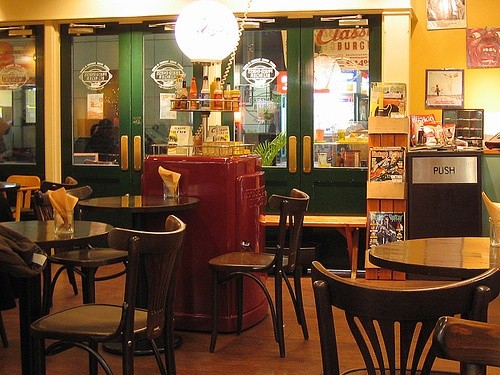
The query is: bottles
[334,153,341,166]
[417,126,425,144]
[446,129,452,144]
[224,82,232,110]
[213,77,223,110]
[189,77,198,108]
[200,76,210,110]
[178,81,188,109]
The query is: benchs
[262,212,367,279]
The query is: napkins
[481,190,500,220]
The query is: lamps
[174,0,240,65]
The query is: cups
[337,130,346,141]
[489,216,500,248]
[316,128,324,143]
[232,89,240,110]
[318,153,327,167]
[53,210,75,233]
[163,182,180,198]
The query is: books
[370,147,405,183]
[209,125,230,143]
[167,126,192,156]
[370,82,407,118]
[370,211,405,249]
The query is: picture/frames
[423,67,466,110]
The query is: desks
[0,218,114,375]
[0,181,21,223]
[79,195,200,358]
[368,235,500,279]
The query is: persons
[84,118,114,162]
[0,117,11,163]
[370,216,403,249]
[337,147,346,167]
[382,152,399,172]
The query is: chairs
[33,187,128,305]
[430,316,500,375]
[22,176,79,222]
[28,213,187,375]
[310,258,500,375]
[5,175,42,223]
[208,188,311,358]
